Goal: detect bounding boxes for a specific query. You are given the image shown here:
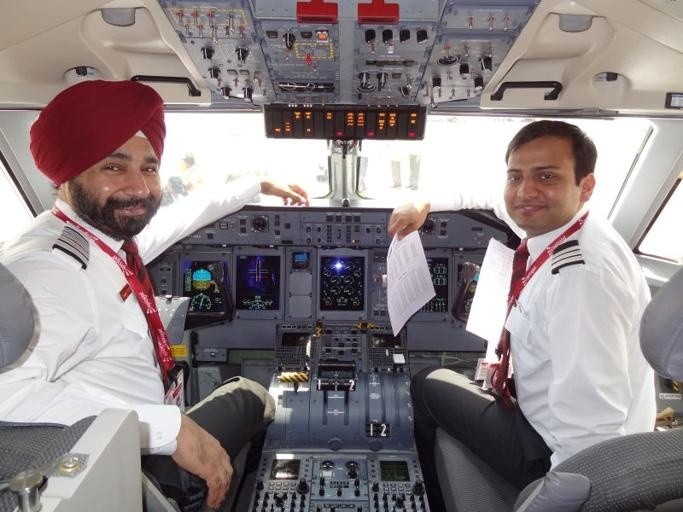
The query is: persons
[0,79,310,512]
[387,120,658,512]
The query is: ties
[487,239,530,409]
[122,239,154,300]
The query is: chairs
[0,263,180,512]
[432,255,681,512]
